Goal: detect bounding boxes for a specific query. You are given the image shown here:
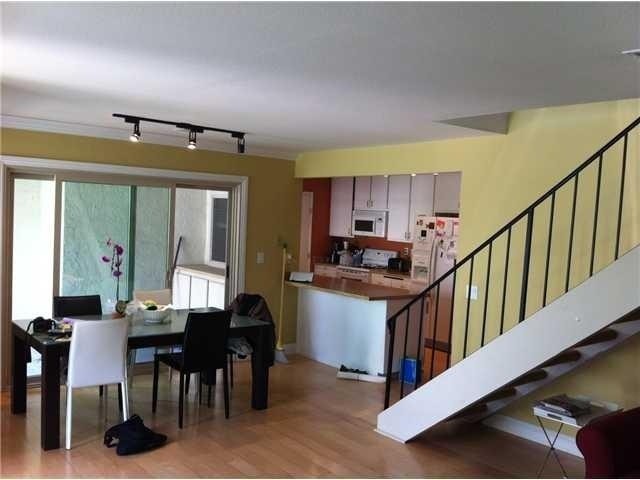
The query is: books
[532,395,608,428]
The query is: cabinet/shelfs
[387,175,434,243]
[355,175,389,212]
[328,176,354,238]
[434,172,460,215]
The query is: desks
[8,304,276,452]
[533,410,616,478]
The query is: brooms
[275,242,290,365]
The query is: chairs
[51,295,276,451]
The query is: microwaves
[351,209,387,238]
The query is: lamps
[128,121,247,154]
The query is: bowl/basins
[138,303,171,324]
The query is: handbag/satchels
[104,414,166,455]
